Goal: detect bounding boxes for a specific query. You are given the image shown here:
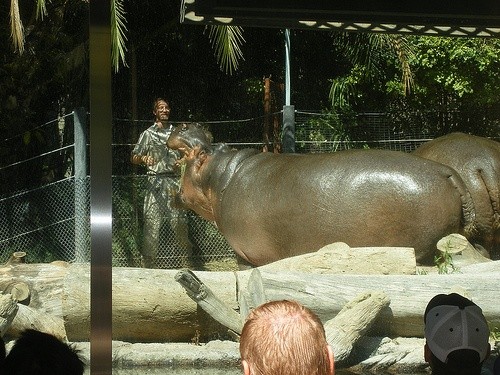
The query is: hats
[424,293,489,366]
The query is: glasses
[154,107,171,112]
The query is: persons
[0,329,86,375]
[129,97,193,270]
[423,293,491,375]
[239,299,334,375]
